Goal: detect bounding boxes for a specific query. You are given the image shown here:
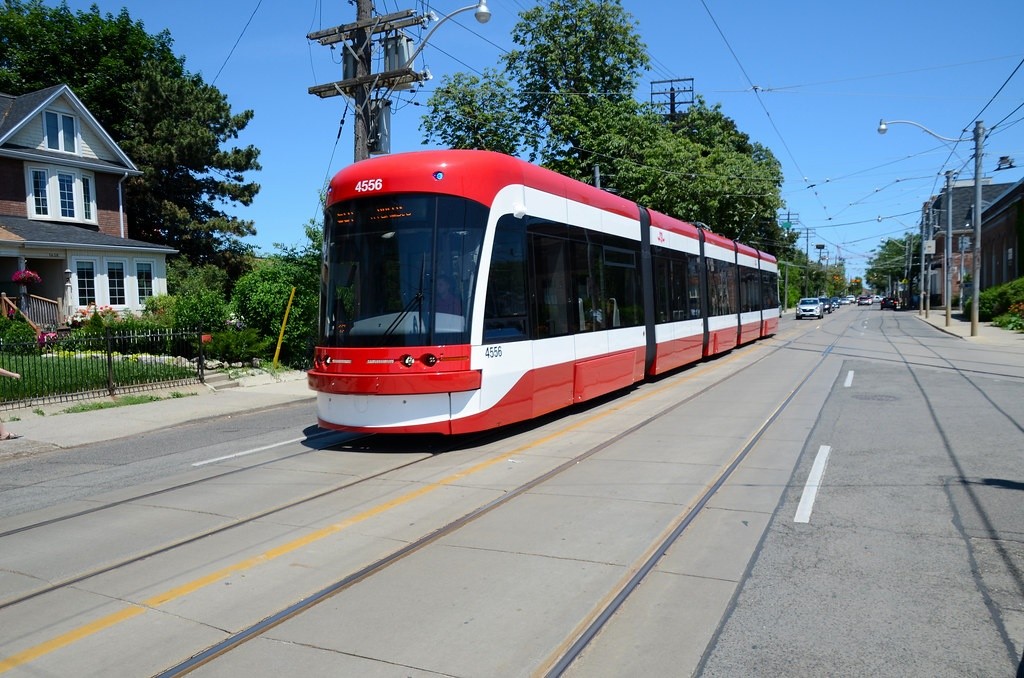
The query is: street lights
[349,0,493,163]
[878,120,986,336]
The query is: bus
[308,148,782,437]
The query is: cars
[881,297,897,311]
[795,297,825,319]
[829,294,883,312]
[820,297,833,314]
